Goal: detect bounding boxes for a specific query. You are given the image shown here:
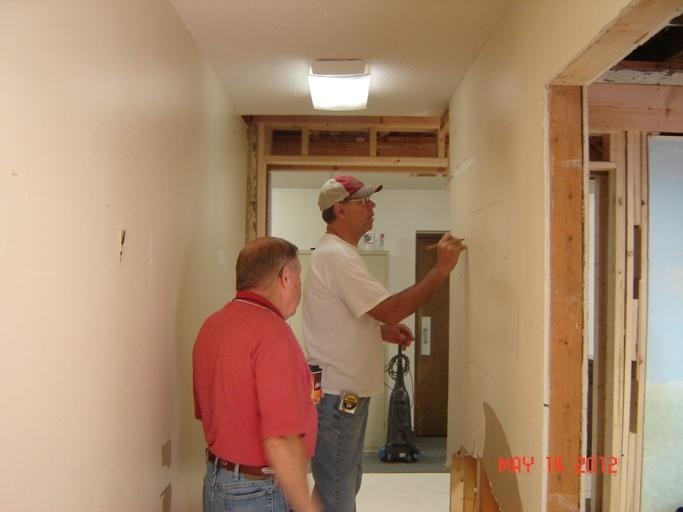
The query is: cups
[308,363,323,406]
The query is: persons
[190,236,325,512]
[297,173,471,512]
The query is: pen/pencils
[426,238,465,252]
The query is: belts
[206,451,276,478]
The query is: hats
[316,174,384,211]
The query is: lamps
[306,57,373,110]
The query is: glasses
[346,195,370,206]
